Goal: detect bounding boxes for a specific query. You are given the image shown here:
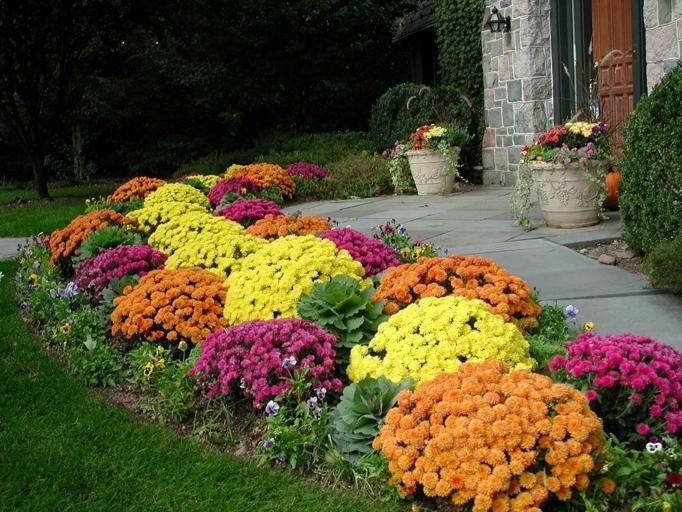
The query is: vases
[528,161,610,229]
[405,147,459,196]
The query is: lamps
[484,5,511,34]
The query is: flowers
[510,121,616,233]
[391,124,468,195]
